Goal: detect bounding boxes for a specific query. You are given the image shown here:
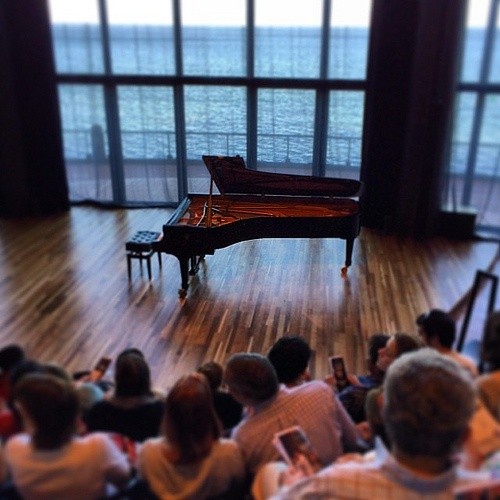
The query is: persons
[251,350,486,500]
[142,371,246,500]
[0,305,499,483]
[3,375,130,500]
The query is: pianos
[149,153,364,299]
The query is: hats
[415,308,456,347]
[114,348,151,390]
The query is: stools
[125,230,164,281]
